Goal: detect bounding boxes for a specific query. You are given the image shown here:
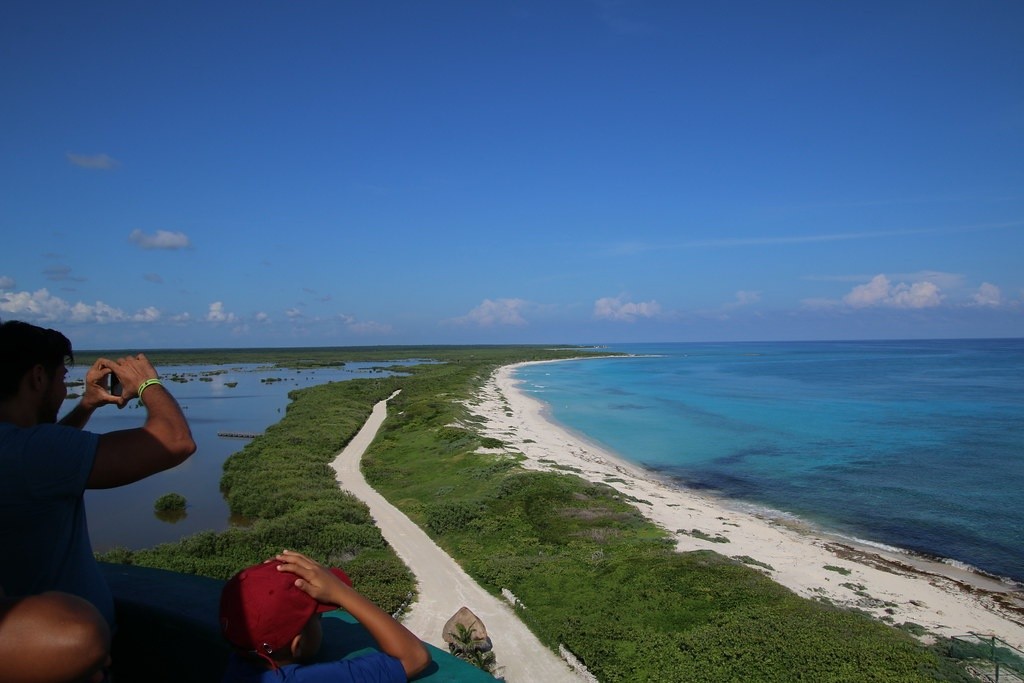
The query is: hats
[219,560,352,676]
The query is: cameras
[110,371,140,399]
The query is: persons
[0,321,197,621]
[218,549,432,683]
[0,590,111,683]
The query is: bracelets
[138,379,162,399]
